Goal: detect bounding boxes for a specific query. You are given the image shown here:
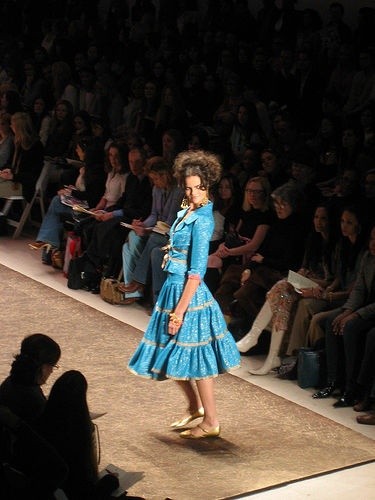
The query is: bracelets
[169,313,183,326]
[329,293,333,303]
[327,286,333,291]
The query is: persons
[0,333,146,500]
[0,0,374,428]
[127,151,240,439]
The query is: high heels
[235,333,258,353]
[249,356,281,376]
[28,241,52,253]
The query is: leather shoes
[354,393,375,412]
[180,424,220,439]
[170,406,205,427]
[117,280,144,294]
[356,412,375,424]
[120,298,143,304]
[333,390,361,407]
[313,382,336,398]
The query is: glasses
[51,363,59,369]
[245,189,264,194]
[274,201,289,209]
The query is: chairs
[0,160,49,239]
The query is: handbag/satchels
[100,277,121,304]
[63,231,81,277]
[67,250,96,289]
[298,345,328,388]
